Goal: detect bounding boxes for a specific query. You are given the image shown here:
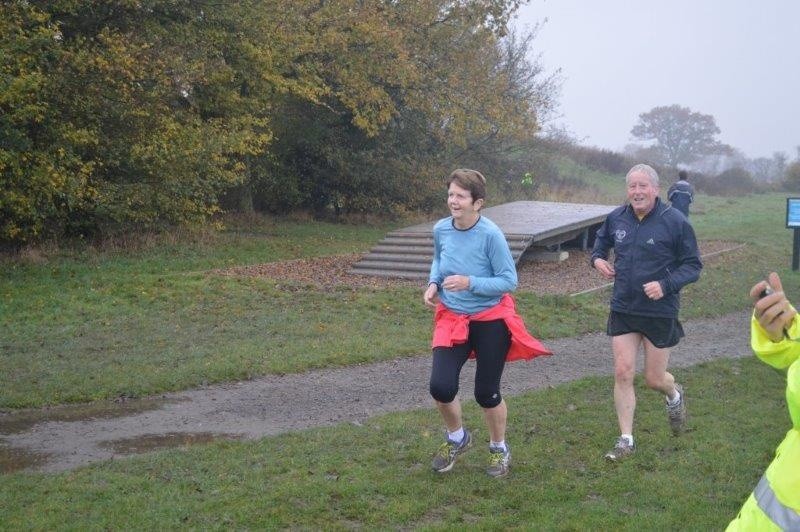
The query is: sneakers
[432,429,472,473]
[666,387,684,433]
[605,437,636,462]
[486,444,512,478]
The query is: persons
[667,170,694,218]
[589,164,703,465]
[423,167,519,481]
[724,271,800,532]
